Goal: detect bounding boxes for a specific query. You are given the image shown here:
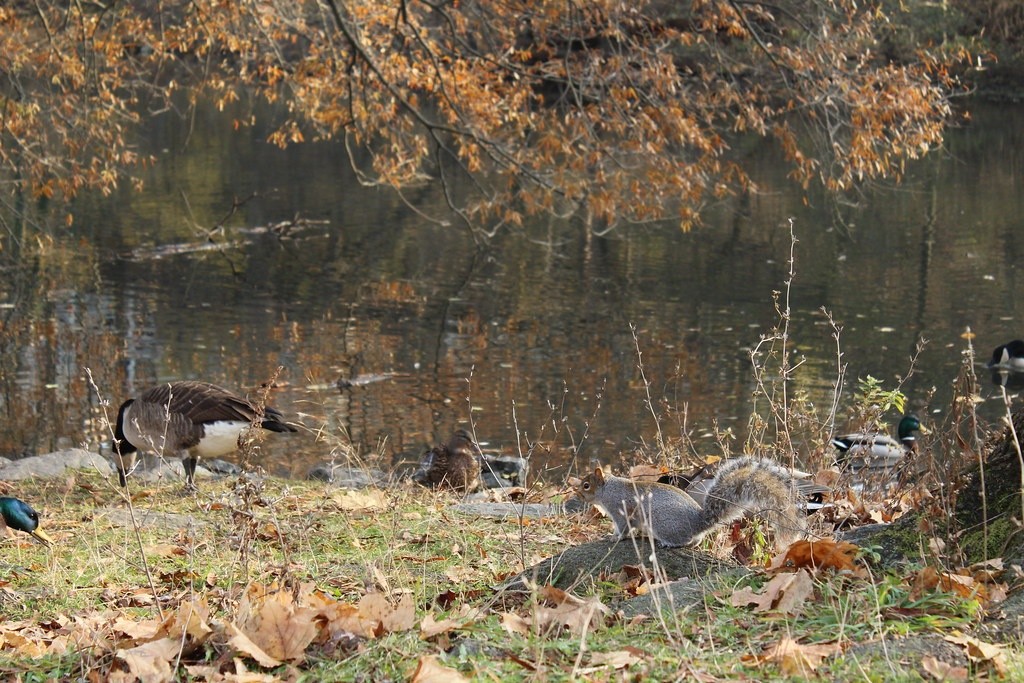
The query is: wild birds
[0,496,56,549]
[112,379,299,489]
[414,429,481,492]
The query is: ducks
[989,369,1024,394]
[987,339,1024,369]
[830,412,933,468]
